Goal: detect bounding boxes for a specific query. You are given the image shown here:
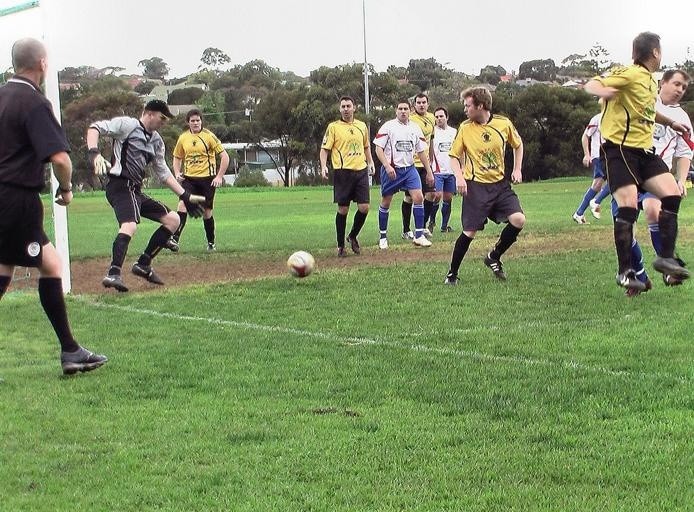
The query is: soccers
[288,249,314,278]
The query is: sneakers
[445,271,460,285]
[484,252,506,278]
[207,241,216,252]
[379,237,388,249]
[101,275,129,291]
[401,221,453,247]
[338,236,360,257]
[589,199,600,219]
[164,235,179,251]
[133,262,164,284]
[573,212,590,224]
[61,346,108,374]
[616,256,689,296]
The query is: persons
[596,67,694,297]
[318,95,377,260]
[571,97,610,225]
[163,109,232,254]
[82,97,205,292]
[442,85,526,285]
[583,30,692,293]
[399,95,437,241]
[0,38,109,376]
[371,100,433,252]
[426,107,458,234]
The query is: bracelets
[58,182,73,193]
[668,120,677,128]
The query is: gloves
[180,190,206,218]
[89,148,112,175]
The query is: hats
[145,100,173,117]
